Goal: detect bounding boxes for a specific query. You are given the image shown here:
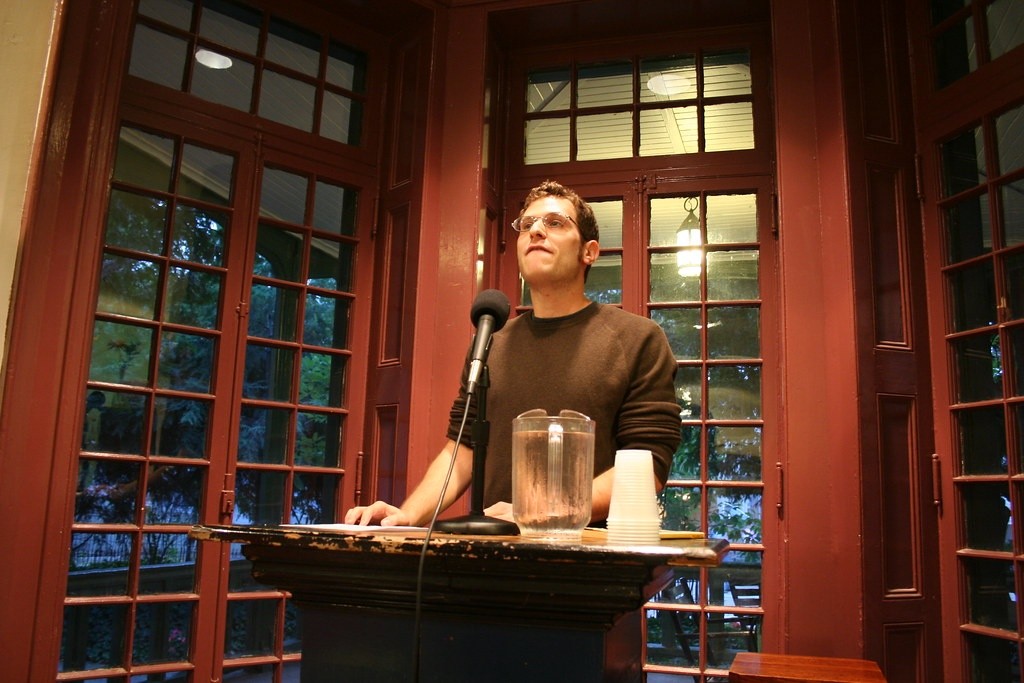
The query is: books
[580,526,705,539]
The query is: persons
[343,182,682,527]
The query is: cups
[607,449,661,547]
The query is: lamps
[675,195,709,277]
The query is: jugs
[511,408,594,542]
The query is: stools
[727,650,889,683]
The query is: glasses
[511,213,589,241]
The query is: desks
[659,562,764,683]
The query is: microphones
[466,288,511,395]
